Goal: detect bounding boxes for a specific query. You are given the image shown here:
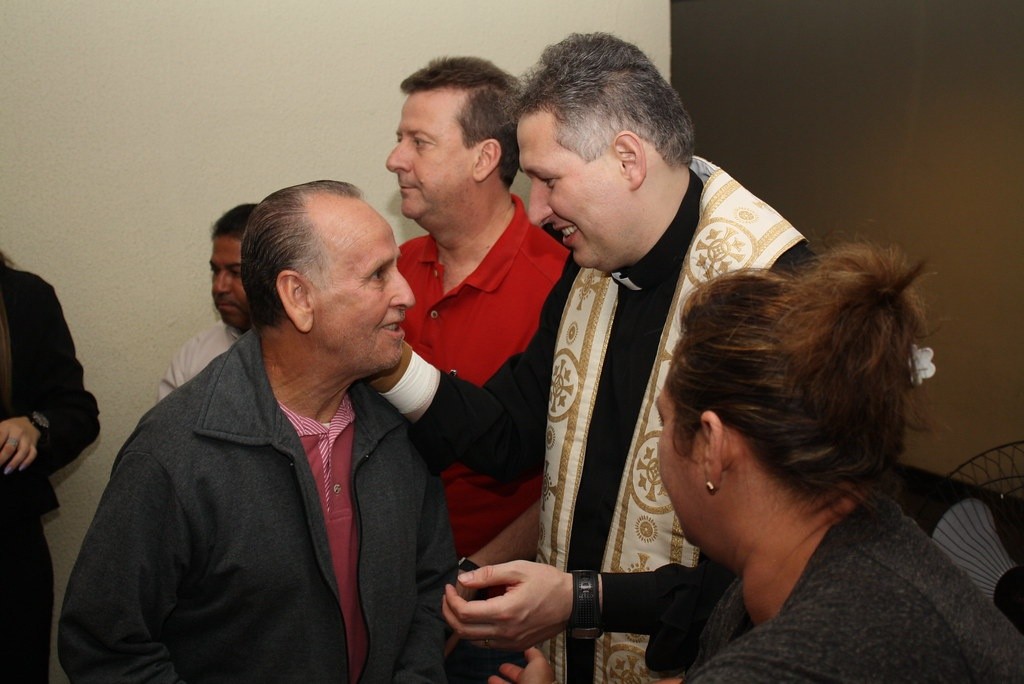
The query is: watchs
[567,568,603,641]
[23,410,49,440]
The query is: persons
[0,252,101,684]
[158,203,256,398]
[362,31,820,684]
[486,245,1024,684]
[57,180,458,684]
[387,56,573,684]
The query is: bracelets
[377,348,437,415]
[457,556,490,599]
